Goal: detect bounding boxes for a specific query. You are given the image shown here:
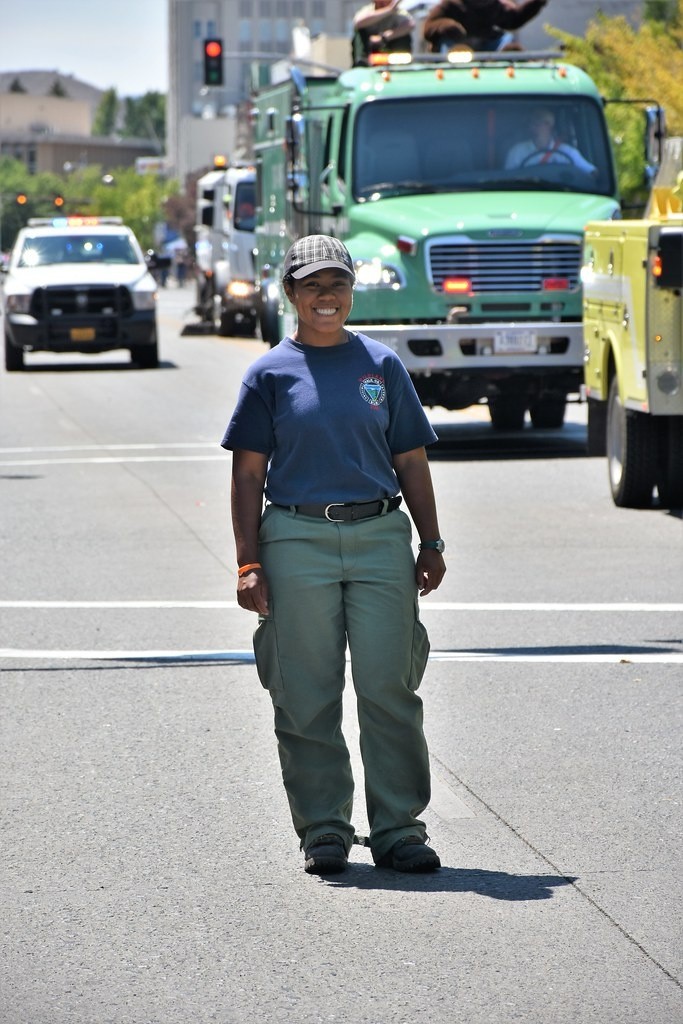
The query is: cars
[0,215,160,372]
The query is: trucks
[254,49,665,418]
[579,136,683,511]
[195,163,265,337]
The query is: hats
[282,234,355,280]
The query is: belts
[276,496,402,523]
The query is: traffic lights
[203,35,223,87]
[13,191,29,209]
[51,190,64,211]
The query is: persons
[147,250,188,288]
[352,0,416,68]
[506,107,601,181]
[425,0,549,54]
[221,235,446,875]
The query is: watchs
[418,538,445,553]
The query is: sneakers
[384,836,441,871]
[303,834,348,872]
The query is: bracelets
[238,562,262,576]
